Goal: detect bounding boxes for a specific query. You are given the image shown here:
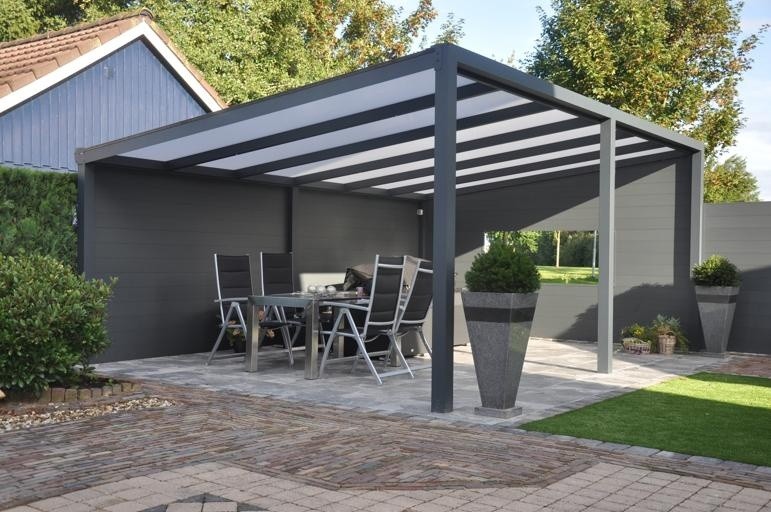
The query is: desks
[245,289,370,379]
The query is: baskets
[621,338,650,353]
[656,335,676,355]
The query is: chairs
[315,251,435,384]
[201,251,326,367]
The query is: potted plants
[693,254,742,351]
[458,234,542,417]
[621,312,691,355]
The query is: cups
[356,287,363,296]
